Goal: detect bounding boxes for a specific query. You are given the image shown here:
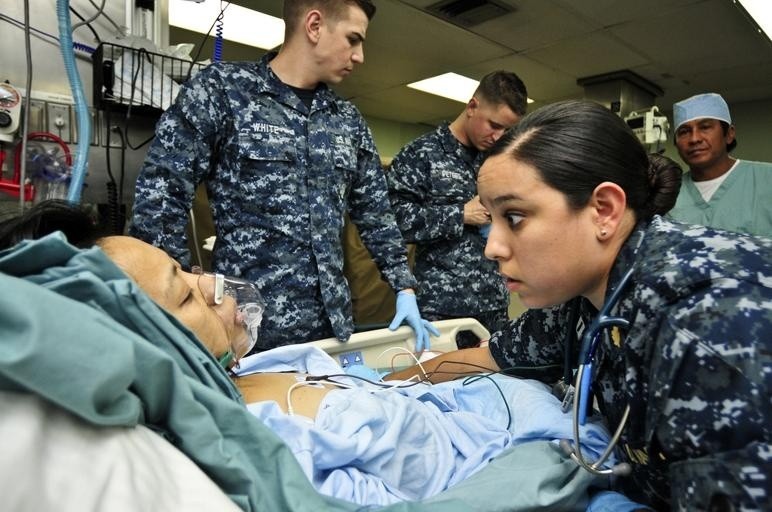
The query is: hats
[673,90,733,132]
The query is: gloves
[388,289,442,352]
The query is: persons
[655,92,771,243]
[128,0,439,357]
[2,198,606,502]
[381,98,772,512]
[384,69,528,333]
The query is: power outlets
[43,100,73,146]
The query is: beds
[2,314,495,511]
